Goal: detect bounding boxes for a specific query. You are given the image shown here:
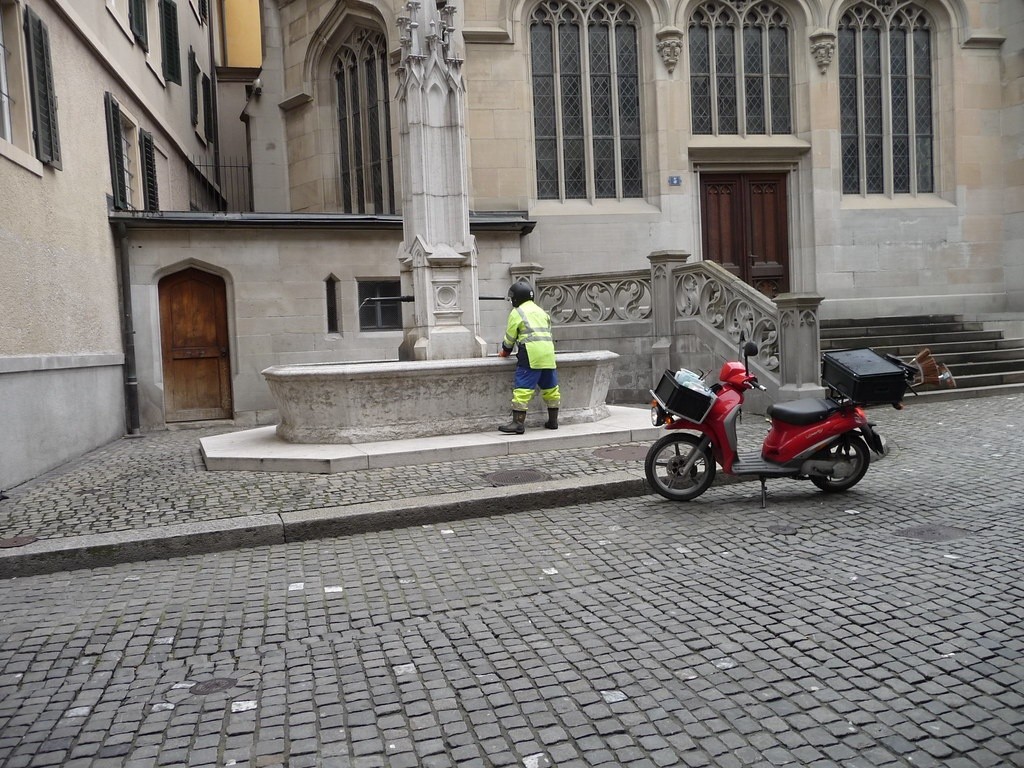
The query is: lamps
[253,78,263,95]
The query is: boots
[544,407,559,429]
[498,410,527,434]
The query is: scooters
[644,329,886,502]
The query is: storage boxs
[820,347,906,405]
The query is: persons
[498,282,561,434]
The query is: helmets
[508,281,535,308]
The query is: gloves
[498,349,511,357]
